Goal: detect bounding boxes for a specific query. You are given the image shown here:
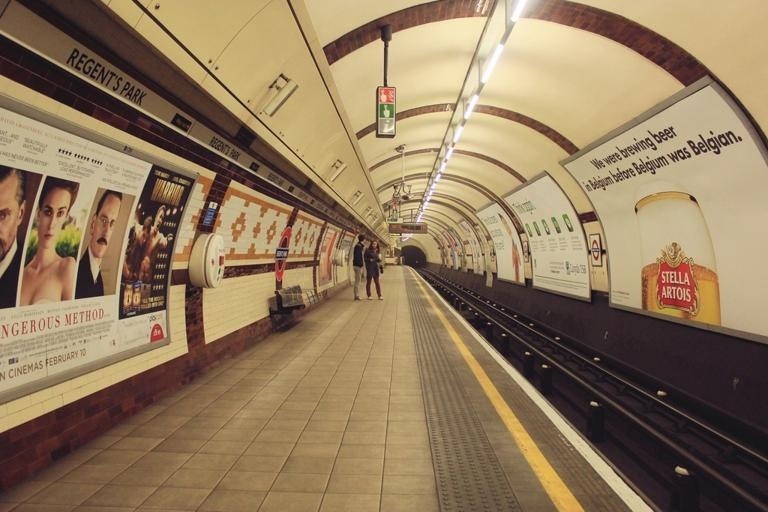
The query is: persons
[125,214,153,280]
[138,204,174,284]
[0,166,26,308]
[364,240,384,299]
[17,176,80,307]
[352,234,367,300]
[74,190,125,300]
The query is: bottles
[634,191,723,328]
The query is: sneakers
[353,295,362,301]
[367,296,373,300]
[376,296,383,300]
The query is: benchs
[269,283,306,330]
